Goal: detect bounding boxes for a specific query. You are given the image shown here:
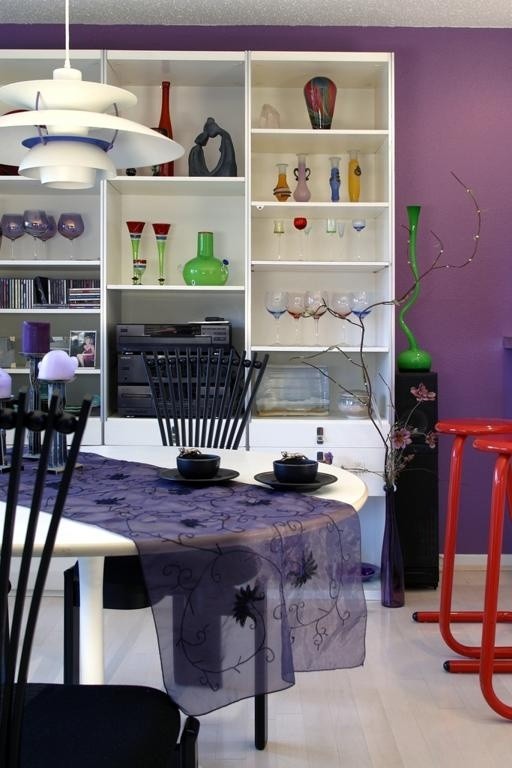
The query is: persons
[77,336,96,367]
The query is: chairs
[60,347,269,751]
[0,392,201,768]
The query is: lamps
[0,0,186,190]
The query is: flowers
[288,172,480,493]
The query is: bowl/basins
[177,453,220,479]
[273,459,318,484]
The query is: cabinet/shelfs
[0,49,396,602]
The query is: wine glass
[286,294,311,347]
[265,291,289,347]
[304,290,329,348]
[57,212,84,260]
[331,292,355,349]
[350,290,373,348]
[0,209,57,260]
[133,259,147,284]
[272,216,367,261]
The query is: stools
[413,417,512,721]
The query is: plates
[159,468,239,489]
[254,471,338,494]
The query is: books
[187,320,230,324]
[1,276,101,310]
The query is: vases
[381,486,404,608]
